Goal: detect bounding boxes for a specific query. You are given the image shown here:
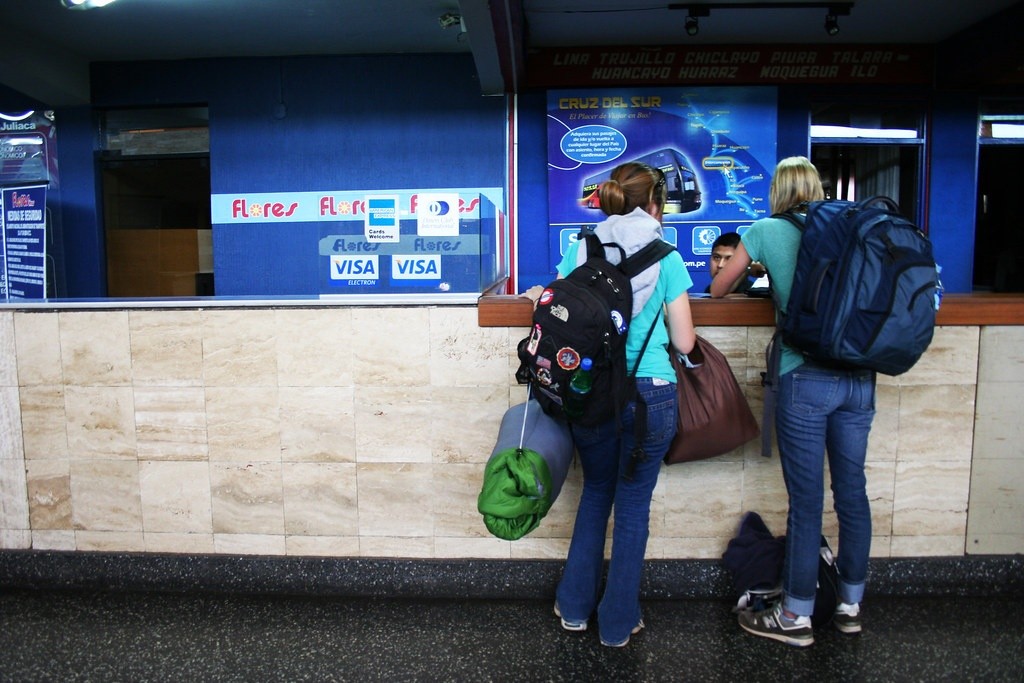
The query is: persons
[518,161,696,649]
[710,155,877,647]
[703,231,742,293]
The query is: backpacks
[525,230,676,479]
[770,194,937,376]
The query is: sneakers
[834,602,862,633]
[737,599,815,647]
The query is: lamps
[823,13,841,36]
[684,14,699,37]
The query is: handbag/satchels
[664,334,762,464]
[478,397,575,541]
[718,511,839,620]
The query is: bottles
[934,262,945,312]
[565,357,592,417]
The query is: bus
[582,149,700,213]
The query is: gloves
[520,285,544,302]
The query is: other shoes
[554,599,588,631]
[600,619,645,647]
